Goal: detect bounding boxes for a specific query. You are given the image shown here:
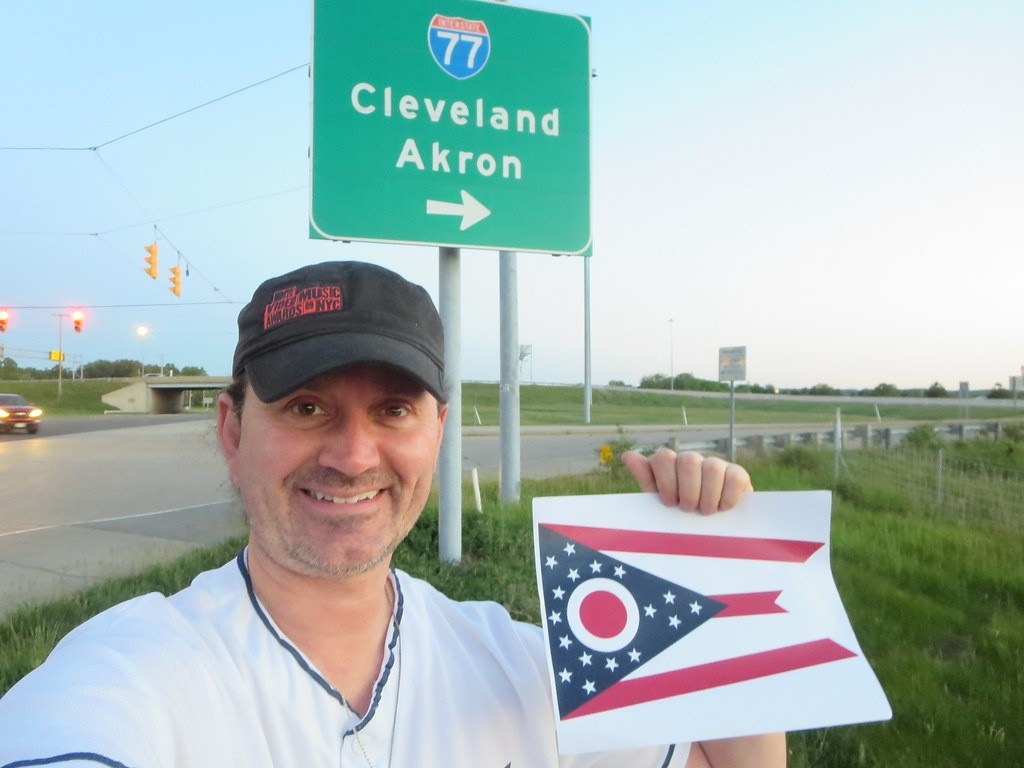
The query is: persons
[0,261,787,768]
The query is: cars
[0,393,43,435]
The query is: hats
[232,261,446,403]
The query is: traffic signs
[308,0,595,261]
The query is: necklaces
[339,587,401,768]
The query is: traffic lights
[72,310,81,335]
[142,242,159,279]
[169,266,180,296]
[0,307,7,330]
